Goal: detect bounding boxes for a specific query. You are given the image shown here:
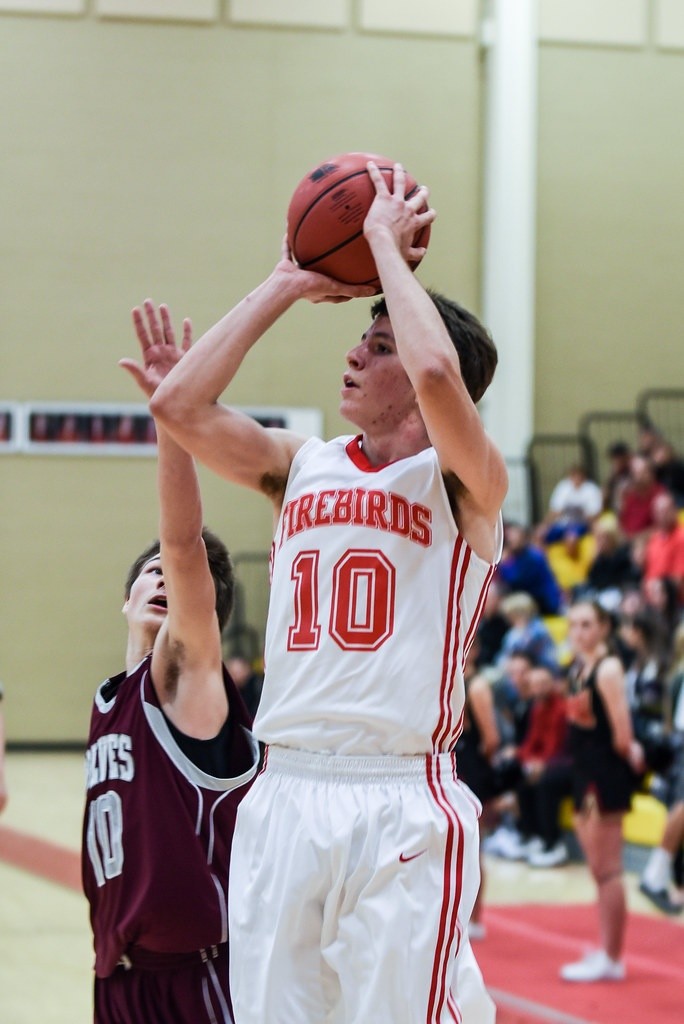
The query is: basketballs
[284,152,431,297]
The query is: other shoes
[468,922,486,937]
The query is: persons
[455,431,684,980]
[150,164,509,1024]
[80,300,259,1024]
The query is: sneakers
[529,838,568,866]
[484,827,512,848]
[560,949,624,980]
[503,830,544,857]
